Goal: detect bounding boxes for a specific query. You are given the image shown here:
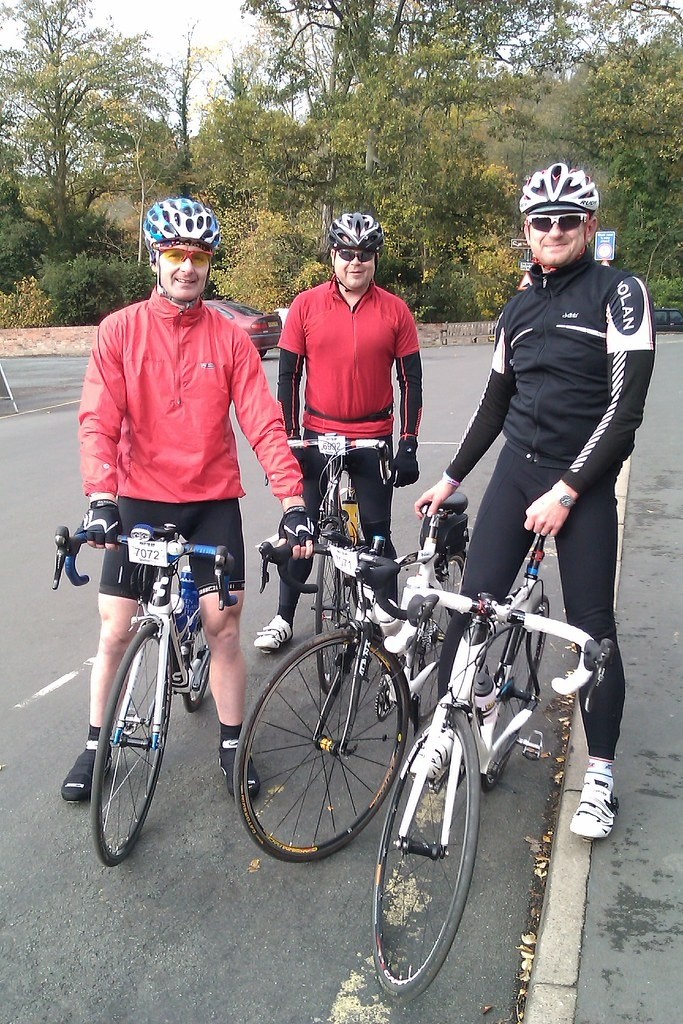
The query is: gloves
[390,436,419,488]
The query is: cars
[201,300,282,361]
[654,308,683,333]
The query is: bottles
[377,598,404,637]
[472,673,496,717]
[342,496,358,546]
[399,576,426,611]
[171,565,199,643]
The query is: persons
[254,212,423,651]
[61,197,315,803]
[414,162,656,838]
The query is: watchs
[552,484,575,510]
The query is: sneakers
[374,590,398,626]
[219,739,260,797]
[570,784,614,838]
[409,724,453,781]
[254,615,293,649]
[62,745,112,801]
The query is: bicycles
[368,527,618,1004]
[229,488,476,865]
[47,522,240,870]
[277,430,397,697]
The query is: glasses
[337,250,375,262]
[159,250,210,267]
[525,213,591,232]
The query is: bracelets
[446,478,459,487]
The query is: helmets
[142,199,222,252]
[519,163,600,211]
[327,212,385,252]
